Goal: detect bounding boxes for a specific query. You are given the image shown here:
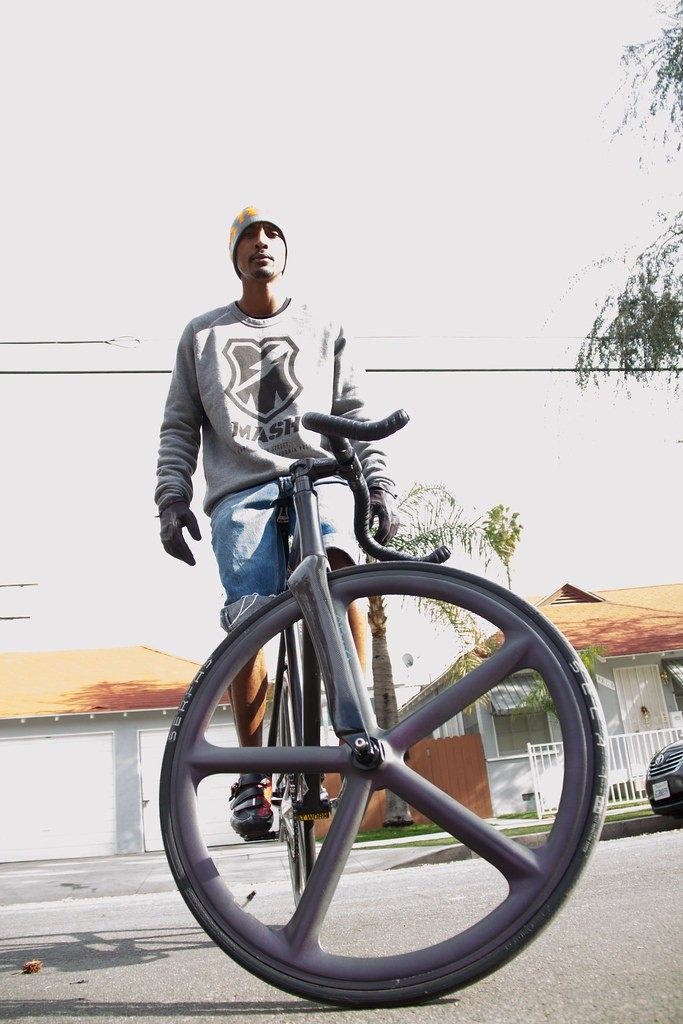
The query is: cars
[226,769,281,841]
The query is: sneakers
[228,773,274,836]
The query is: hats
[229,205,288,279]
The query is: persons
[155,205,397,842]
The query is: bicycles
[158,409,612,1008]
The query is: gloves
[160,502,202,567]
[368,487,399,546]
